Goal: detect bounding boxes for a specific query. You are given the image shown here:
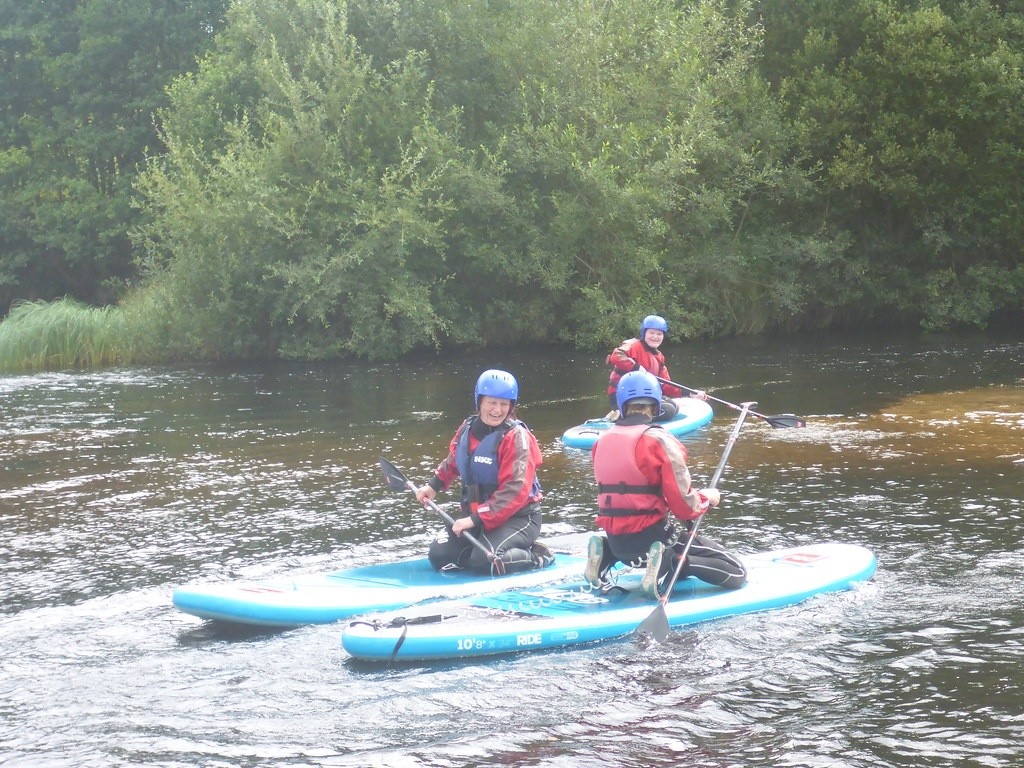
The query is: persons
[585,371,747,603]
[605,315,707,422]
[417,369,556,575]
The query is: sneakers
[582,535,614,589]
[640,541,676,601]
[531,541,556,568]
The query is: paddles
[376,454,507,579]
[624,399,757,642]
[642,368,806,430]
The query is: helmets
[641,315,668,341]
[615,370,663,420]
[474,370,519,414]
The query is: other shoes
[610,410,621,422]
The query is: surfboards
[561,395,717,449]
[342,541,880,661]
[168,536,595,626]
